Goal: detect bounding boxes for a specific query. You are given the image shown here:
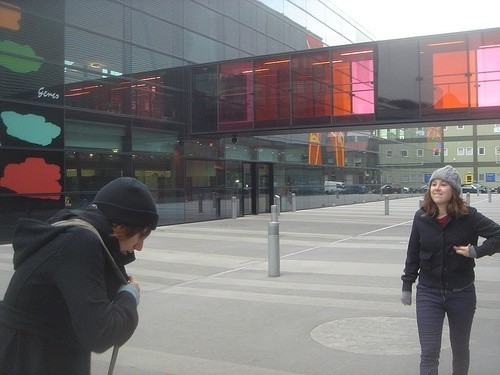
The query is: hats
[429,164,463,194]
[92,176,159,230]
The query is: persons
[1,176,160,374]
[399,164,500,375]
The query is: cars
[324,181,500,194]
